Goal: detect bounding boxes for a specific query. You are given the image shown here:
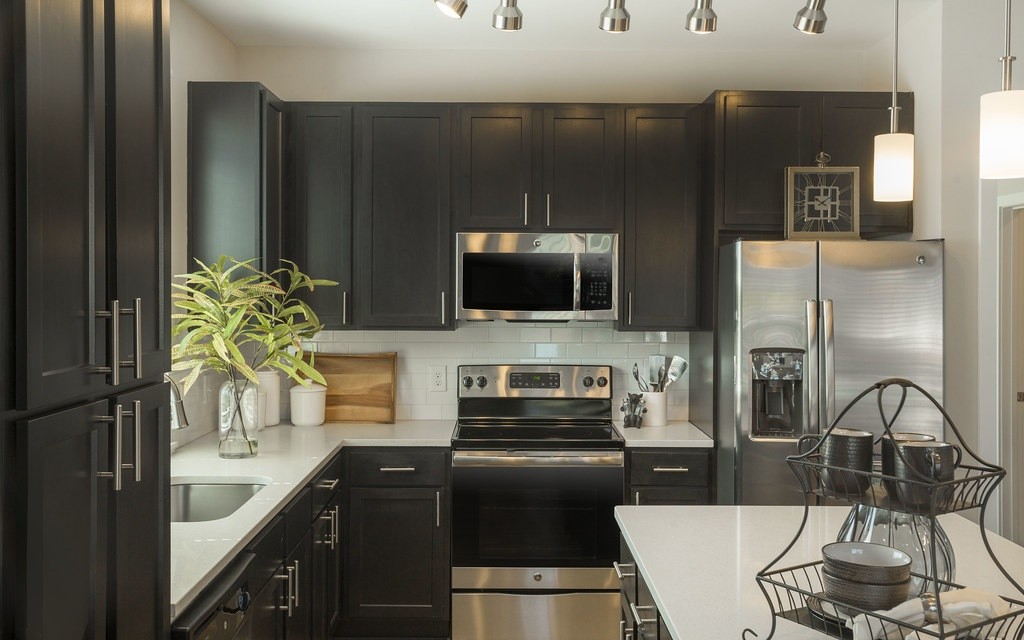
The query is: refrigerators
[716,235,945,505]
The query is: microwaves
[456,231,619,326]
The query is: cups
[821,427,874,495]
[890,441,956,511]
[880,432,936,500]
[641,390,668,426]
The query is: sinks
[169,475,272,522]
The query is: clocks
[784,152,860,241]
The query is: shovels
[664,356,689,391]
[648,354,666,392]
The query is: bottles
[232,366,281,432]
[290,378,327,426]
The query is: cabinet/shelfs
[626,450,711,562]
[186,79,915,330]
[173,447,454,640]
[616,533,673,640]
[0,2,175,640]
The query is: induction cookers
[450,364,625,451]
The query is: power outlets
[429,364,447,393]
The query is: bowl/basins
[821,541,912,585]
[821,566,913,617]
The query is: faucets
[164,373,189,431]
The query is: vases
[219,379,259,460]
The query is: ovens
[449,449,628,640]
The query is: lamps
[491,0,523,31]
[792,0,827,35]
[598,0,630,33]
[977,0,1024,177]
[684,0,717,34]
[434,0,468,19]
[873,3,914,201]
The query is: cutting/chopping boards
[295,352,398,425]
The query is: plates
[807,591,857,633]
